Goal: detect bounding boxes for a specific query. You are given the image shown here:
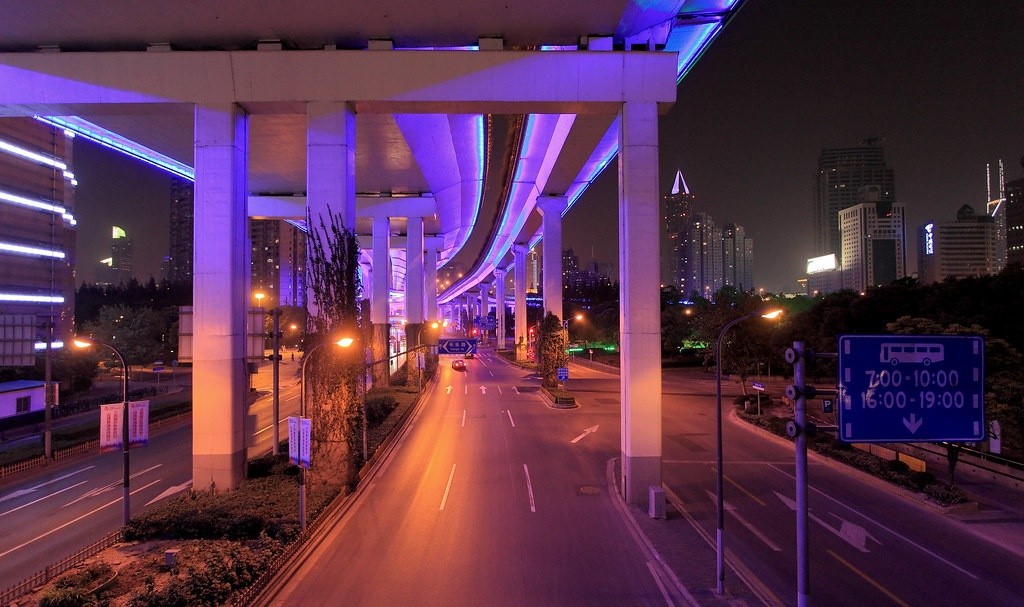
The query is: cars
[451,359,466,370]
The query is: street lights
[73,337,130,525]
[301,337,355,532]
[417,322,438,393]
[714,305,783,595]
[563,314,583,395]
